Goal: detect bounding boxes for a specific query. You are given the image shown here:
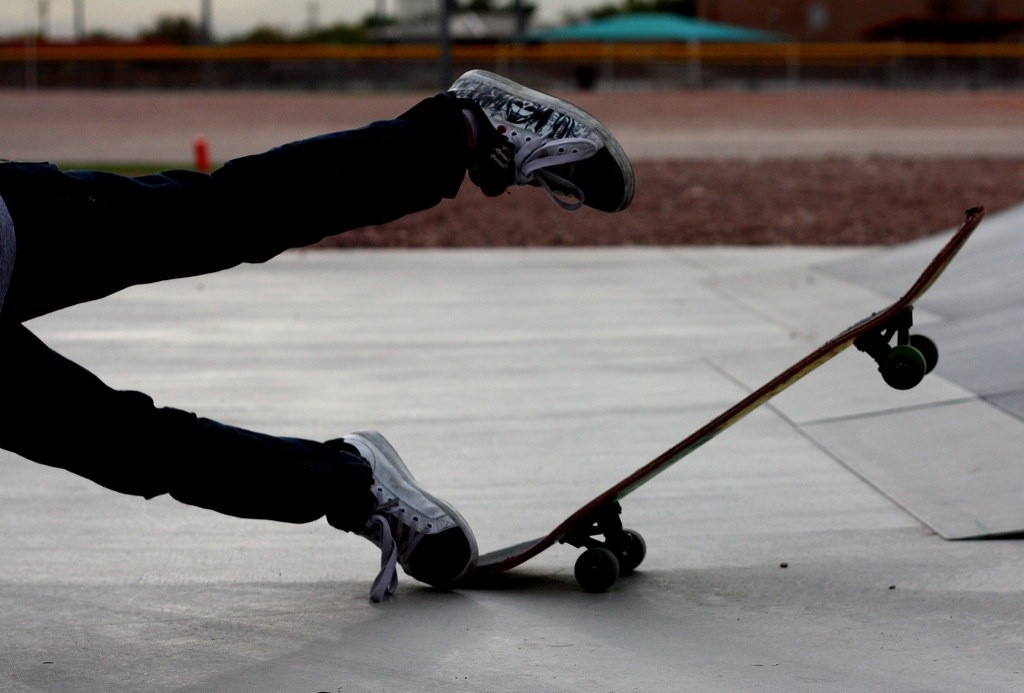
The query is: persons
[0,69,635,604]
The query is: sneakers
[323,429,479,602]
[446,68,635,212]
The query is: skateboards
[443,203,990,593]
